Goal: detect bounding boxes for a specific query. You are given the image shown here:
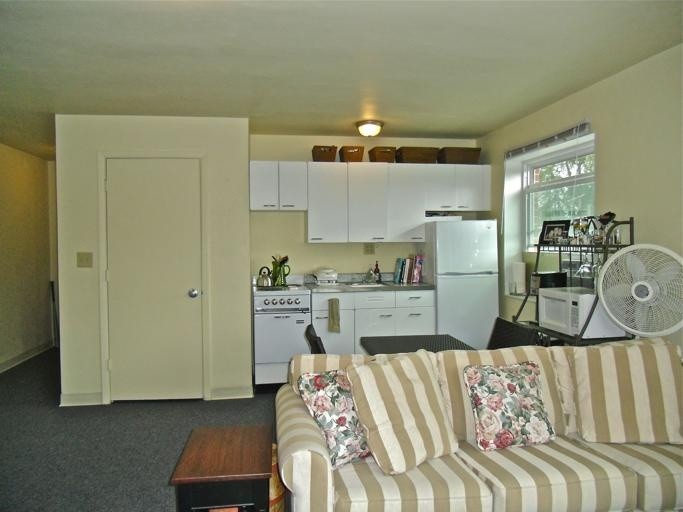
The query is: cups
[548,218,621,246]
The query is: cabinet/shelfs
[248,158,279,212]
[352,285,394,355]
[306,160,347,245]
[386,161,426,245]
[277,160,308,212]
[345,160,391,245]
[394,284,437,336]
[455,163,491,212]
[310,288,353,357]
[508,216,639,344]
[423,163,457,212]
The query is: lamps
[354,118,383,137]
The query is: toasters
[313,267,338,285]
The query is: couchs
[273,336,681,512]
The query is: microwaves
[536,285,629,338]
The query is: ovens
[253,309,311,389]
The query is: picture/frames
[538,218,572,246]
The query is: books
[393,254,423,284]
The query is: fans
[597,243,682,338]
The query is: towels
[327,297,341,335]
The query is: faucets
[365,268,378,282]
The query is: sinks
[349,281,387,288]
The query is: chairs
[303,322,325,355]
[486,316,542,351]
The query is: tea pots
[270,261,290,286]
[256,266,273,286]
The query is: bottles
[374,263,381,283]
[366,265,374,282]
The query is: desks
[357,333,475,355]
[166,423,275,512]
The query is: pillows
[564,342,682,447]
[342,349,460,477]
[296,369,369,472]
[461,360,555,451]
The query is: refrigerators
[432,217,501,350]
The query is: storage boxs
[439,147,482,165]
[394,146,440,163]
